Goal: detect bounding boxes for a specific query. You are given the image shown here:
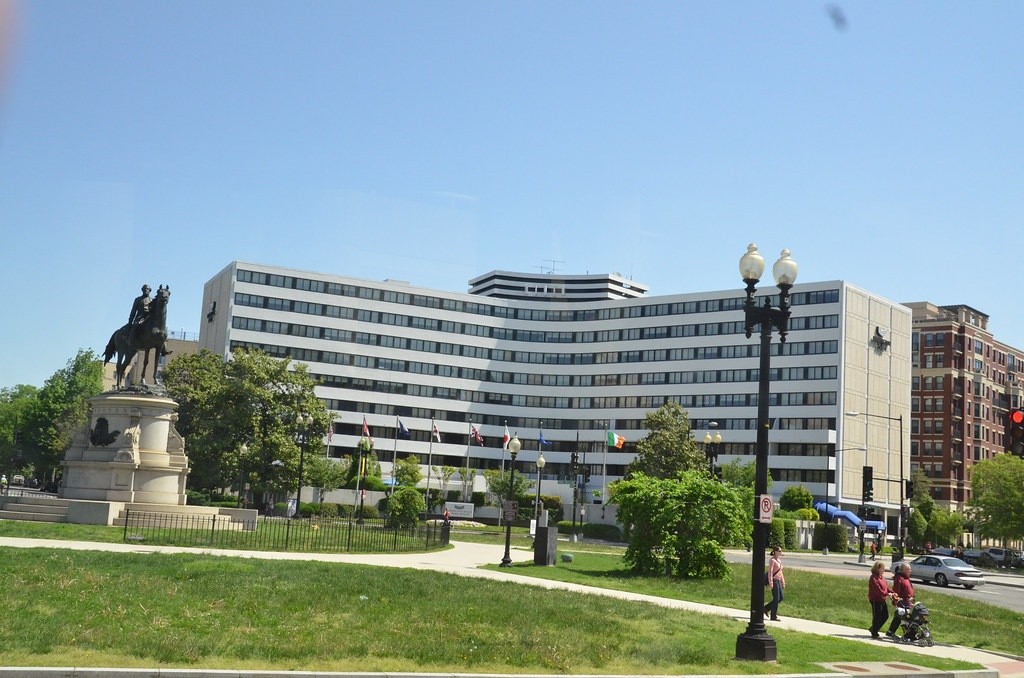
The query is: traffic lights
[1011,408,1024,456]
[857,506,874,519]
[585,466,591,483]
[863,466,872,489]
[906,480,913,499]
[863,486,873,502]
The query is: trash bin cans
[892,553,901,562]
[535,526,559,566]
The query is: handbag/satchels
[764,572,769,586]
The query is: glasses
[775,550,782,552]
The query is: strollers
[889,592,934,647]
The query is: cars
[929,546,1024,569]
[889,554,985,589]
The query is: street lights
[734,242,798,661]
[845,410,904,559]
[237,443,248,509]
[359,436,374,524]
[685,421,719,444]
[292,412,313,519]
[531,452,546,550]
[823,447,868,555]
[704,431,722,478]
[498,432,522,568]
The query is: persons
[886,563,920,639]
[1,475,7,494]
[241,497,248,508]
[444,507,451,523]
[867,562,898,638]
[925,542,931,548]
[125,284,173,356]
[870,540,876,560]
[269,499,274,516]
[764,546,785,621]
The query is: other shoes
[868,628,883,639]
[771,616,781,621]
[886,631,899,639]
[763,607,770,617]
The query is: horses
[102,284,171,389]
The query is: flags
[472,425,483,446]
[607,431,625,449]
[433,423,441,442]
[503,425,510,450]
[329,426,333,441]
[364,419,369,436]
[539,431,552,446]
[399,420,411,436]
